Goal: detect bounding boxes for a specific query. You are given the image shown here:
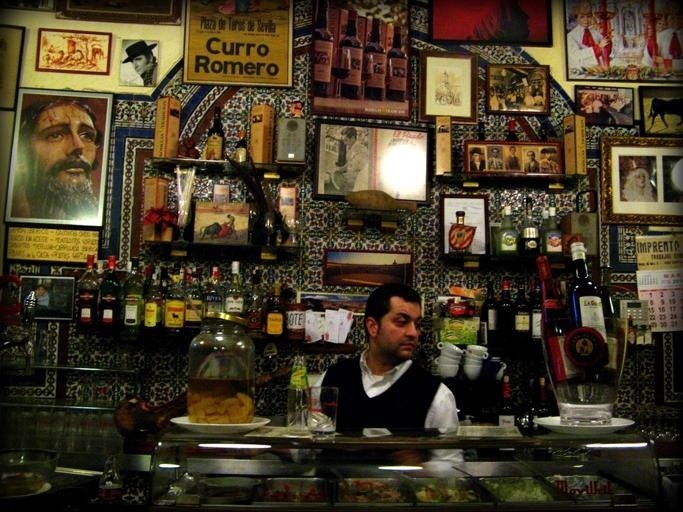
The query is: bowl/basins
[0,447,58,496]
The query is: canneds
[187,310,257,424]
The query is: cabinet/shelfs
[143,155,307,250]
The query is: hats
[123,41,157,63]
[470,148,484,154]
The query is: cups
[538,318,628,426]
[434,340,490,382]
[285,303,304,340]
[307,385,338,439]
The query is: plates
[533,415,635,436]
[0,483,52,499]
[168,413,272,437]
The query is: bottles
[625,63,639,80]
[493,192,564,255]
[497,374,551,436]
[448,211,476,253]
[234,130,247,163]
[598,264,617,375]
[313,2,406,103]
[75,254,286,336]
[287,357,311,434]
[483,277,564,338]
[535,255,572,383]
[626,317,653,347]
[205,106,225,160]
[564,237,609,366]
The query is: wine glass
[282,213,304,248]
[634,405,682,442]
[171,164,196,245]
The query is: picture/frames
[322,248,415,289]
[310,119,435,206]
[426,1,554,46]
[17,274,80,325]
[441,192,490,255]
[462,139,564,175]
[52,0,184,28]
[0,22,28,227]
[485,62,550,116]
[599,137,682,227]
[4,87,115,231]
[34,27,112,75]
[179,1,298,88]
[562,0,681,84]
[416,49,480,126]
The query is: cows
[199,223,222,241]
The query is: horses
[648,98,683,128]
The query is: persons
[621,168,657,202]
[271,282,464,480]
[469,146,560,174]
[12,97,105,219]
[226,215,238,241]
[642,16,683,71]
[325,127,370,194]
[23,286,49,307]
[489,87,546,111]
[566,14,609,68]
[122,41,158,87]
